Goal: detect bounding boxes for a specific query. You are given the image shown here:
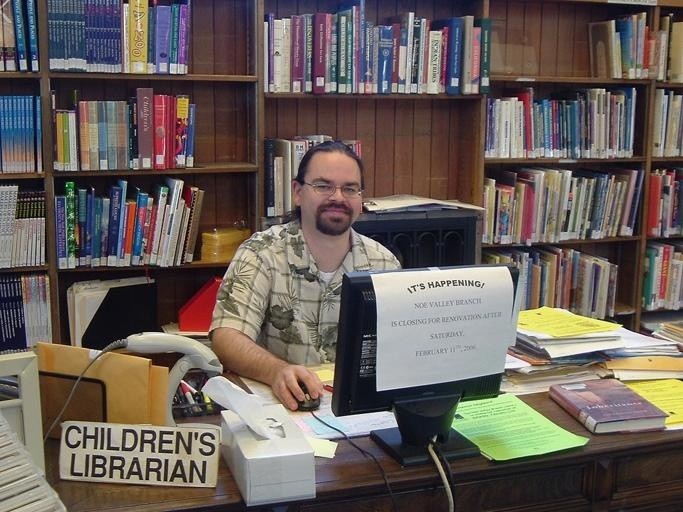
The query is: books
[362,193,487,214]
[260,2,489,97]
[588,10,680,83]
[491,85,683,313]
[498,306,683,395]
[0,1,207,387]
[262,137,363,220]
[548,378,670,437]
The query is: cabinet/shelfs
[480,0,683,345]
[261,2,486,343]
[1,0,261,345]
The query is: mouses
[294,380,320,411]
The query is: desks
[34,323,681,512]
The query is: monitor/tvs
[330,262,520,469]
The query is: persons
[206,137,402,411]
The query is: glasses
[294,177,362,199]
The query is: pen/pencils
[172,376,213,414]
[322,384,333,392]
